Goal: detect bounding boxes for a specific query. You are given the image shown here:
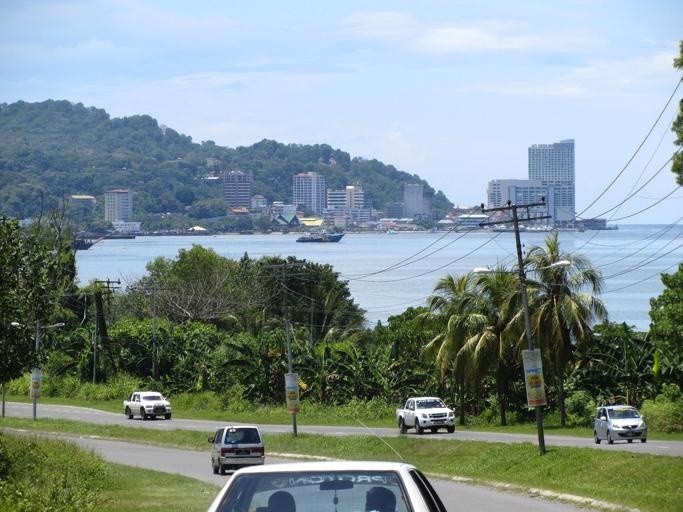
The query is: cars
[205,460,447,512]
[594,404,647,444]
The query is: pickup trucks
[396,396,455,434]
[123,391,171,421]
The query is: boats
[296,234,344,242]
[71,240,92,250]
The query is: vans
[207,426,265,475]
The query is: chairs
[365,486,395,512]
[269,491,296,512]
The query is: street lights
[10,321,65,421]
[473,259,571,457]
